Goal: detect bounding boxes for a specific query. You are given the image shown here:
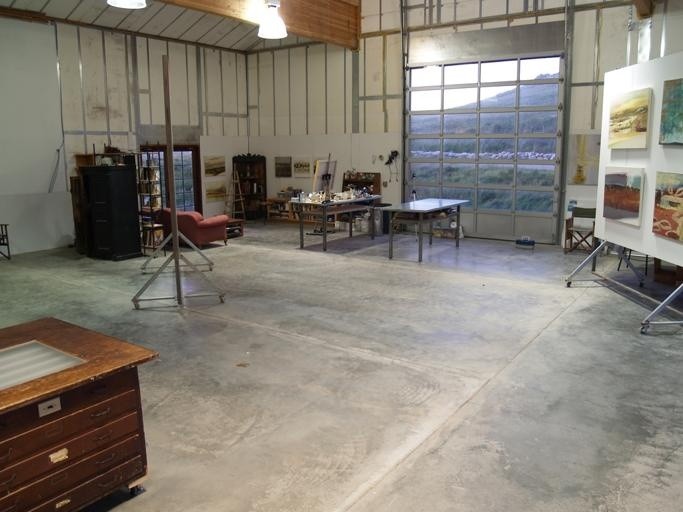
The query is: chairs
[564,206,596,256]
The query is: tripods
[394,191,433,241]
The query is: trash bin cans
[371,203,392,235]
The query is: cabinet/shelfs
[233,152,267,222]
[1,317,161,512]
[339,172,380,223]
[78,163,143,261]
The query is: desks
[379,198,470,262]
[288,194,383,250]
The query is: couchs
[139,205,229,250]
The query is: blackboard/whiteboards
[595,53,683,266]
